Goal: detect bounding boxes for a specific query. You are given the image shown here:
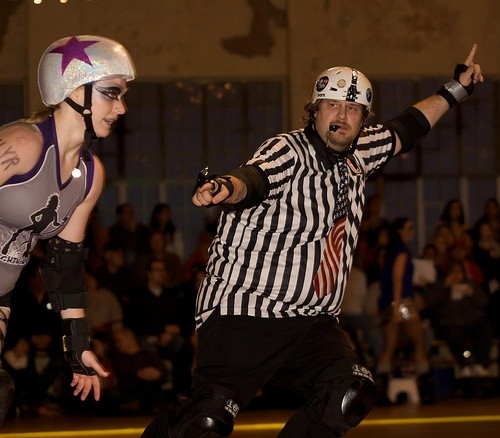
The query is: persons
[0,36,136,399]
[1,206,218,420]
[339,197,500,380]
[139,44,484,438]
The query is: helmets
[36,35,134,106]
[312,67,373,112]
